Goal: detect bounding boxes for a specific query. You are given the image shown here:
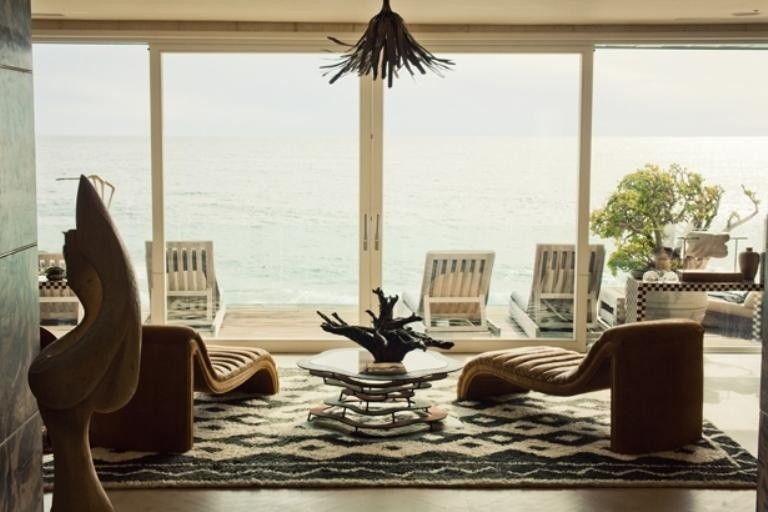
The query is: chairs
[33,251,88,331]
[139,240,228,337]
[508,241,613,338]
[594,274,711,332]
[702,286,762,333]
[88,320,281,455]
[402,251,503,338]
[453,312,706,454]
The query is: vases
[738,247,759,285]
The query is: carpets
[38,348,761,494]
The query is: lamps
[321,1,453,89]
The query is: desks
[295,343,462,440]
